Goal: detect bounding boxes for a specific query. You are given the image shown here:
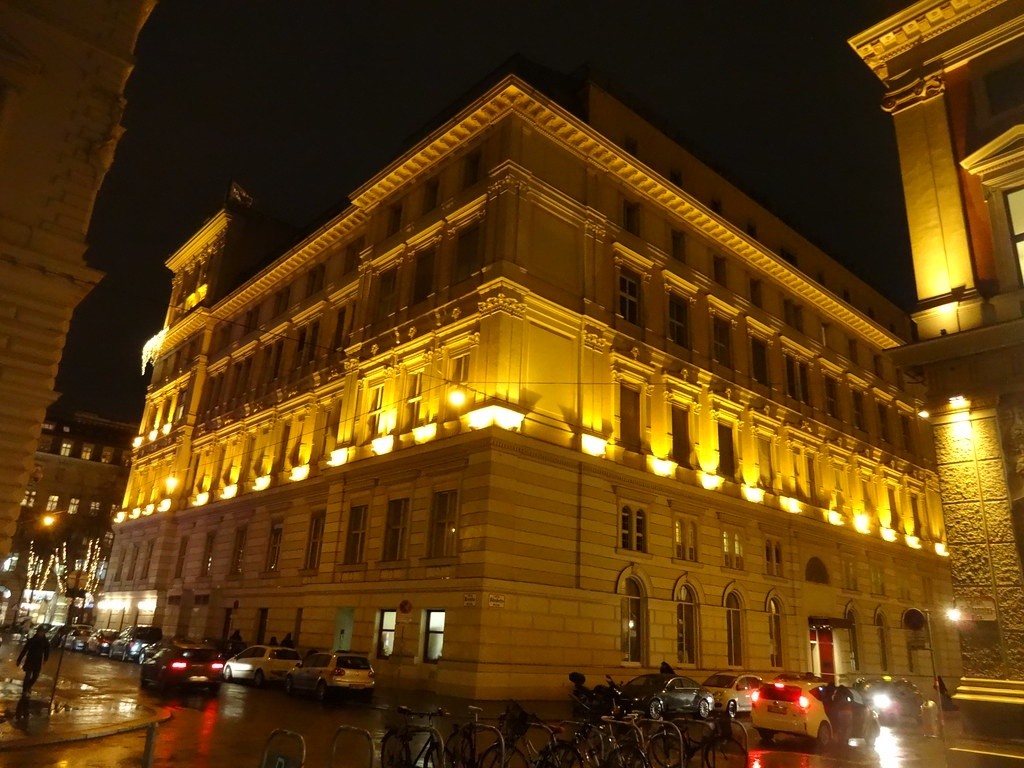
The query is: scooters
[568,671,618,716]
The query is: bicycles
[381,706,750,768]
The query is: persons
[17,625,50,695]
[231,629,242,640]
[269,636,278,645]
[281,632,294,649]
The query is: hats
[34,625,47,631]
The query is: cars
[701,672,764,719]
[222,645,302,687]
[61,630,95,650]
[87,629,119,656]
[141,643,224,697]
[853,675,926,730]
[108,626,162,664]
[616,673,716,721]
[285,651,376,702]
[752,672,880,753]
[27,623,93,649]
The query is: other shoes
[22,689,31,698]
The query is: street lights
[40,512,97,717]
